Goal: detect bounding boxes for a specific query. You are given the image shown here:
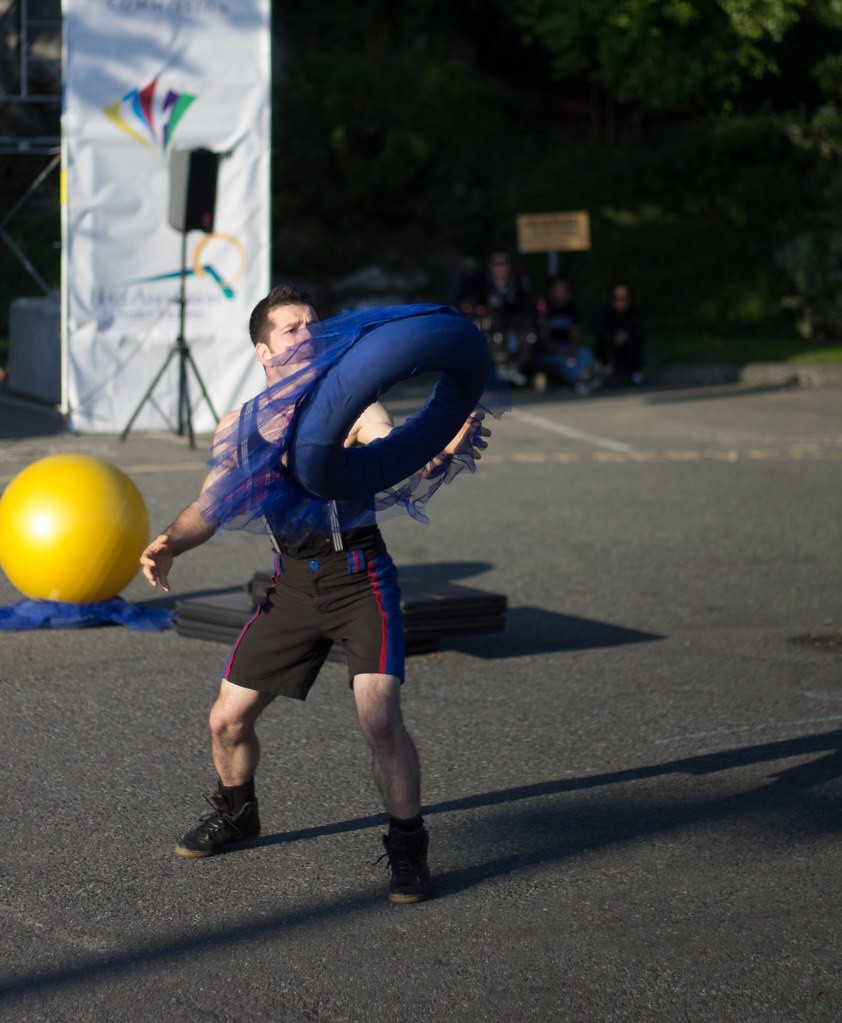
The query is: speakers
[166,147,219,233]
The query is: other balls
[0,452,150,606]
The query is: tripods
[120,233,220,453]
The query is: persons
[140,284,493,904]
[452,257,648,392]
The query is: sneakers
[371,827,433,904]
[175,793,261,858]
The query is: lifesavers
[286,311,496,503]
